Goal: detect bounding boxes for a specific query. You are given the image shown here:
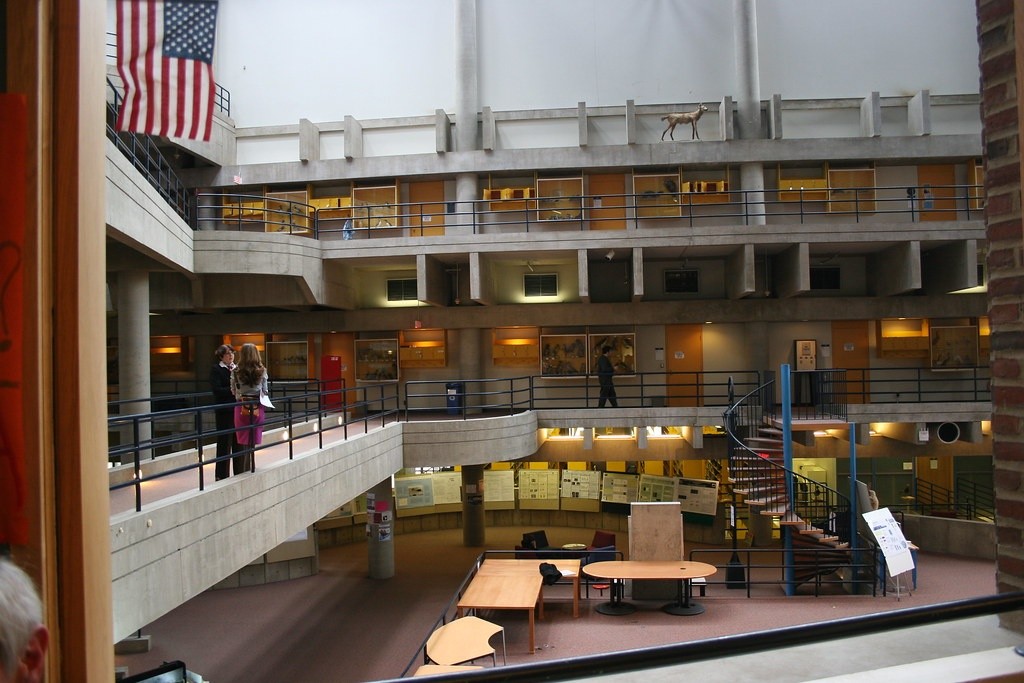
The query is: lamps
[527,260,537,272]
[604,250,615,262]
[764,248,770,296]
[454,264,461,305]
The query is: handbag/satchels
[241,396,260,417]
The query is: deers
[661,102,710,141]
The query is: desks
[582,561,718,616]
[901,495,915,514]
[456,558,581,655]
[859,531,919,591]
[425,615,507,668]
[414,664,484,677]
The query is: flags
[116,0,220,142]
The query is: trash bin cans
[445,384,462,416]
[819,379,834,403]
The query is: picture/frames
[663,269,700,296]
[386,278,418,300]
[810,265,843,292]
[522,273,558,297]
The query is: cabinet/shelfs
[491,327,636,378]
[222,187,265,225]
[266,341,315,384]
[680,164,730,204]
[265,184,315,238]
[825,162,877,215]
[928,319,981,371]
[968,160,984,210]
[353,179,403,229]
[354,339,399,382]
[631,167,681,217]
[308,182,353,219]
[875,319,930,363]
[397,329,448,369]
[483,172,538,211]
[537,170,585,221]
[777,164,830,202]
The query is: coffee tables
[559,543,596,564]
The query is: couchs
[515,530,617,567]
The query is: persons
[0,560,50,683]
[598,346,617,407]
[342,216,355,239]
[210,344,240,481]
[230,344,268,471]
